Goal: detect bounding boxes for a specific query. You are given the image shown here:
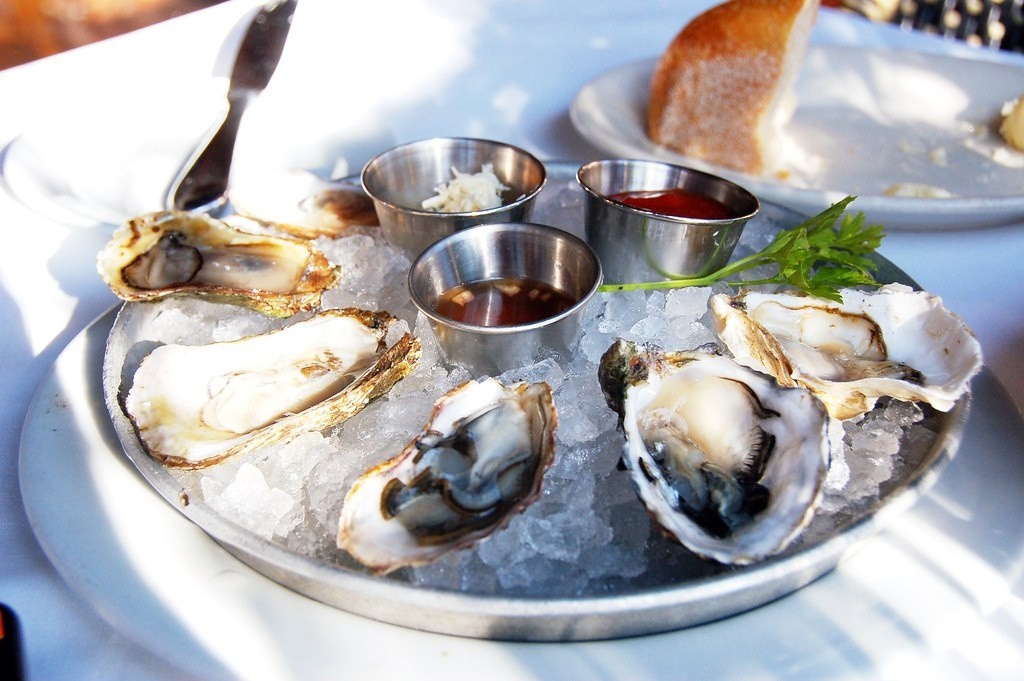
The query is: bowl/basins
[575,159,761,288]
[406,221,603,380]
[101,159,974,643]
[361,135,547,263]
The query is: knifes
[163,0,298,212]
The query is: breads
[646,0,822,180]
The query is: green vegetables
[595,193,887,307]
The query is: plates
[569,43,1024,233]
[17,300,1024,681]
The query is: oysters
[97,157,985,577]
